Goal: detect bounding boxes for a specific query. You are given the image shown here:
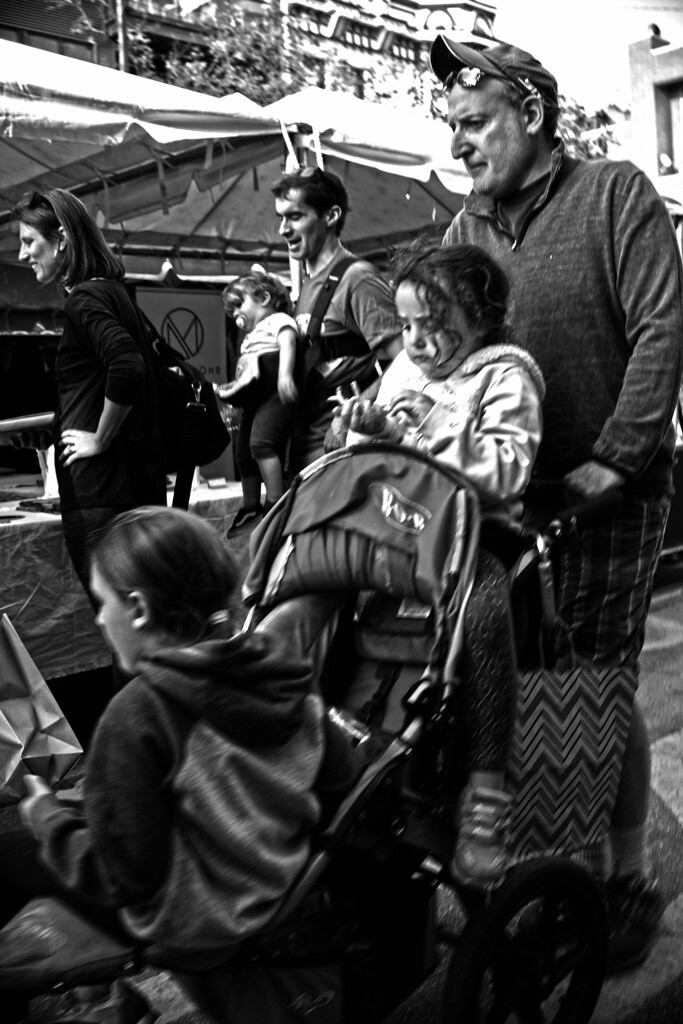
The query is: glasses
[440,65,491,91]
[288,166,325,180]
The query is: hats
[428,34,562,115]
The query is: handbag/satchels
[135,301,228,464]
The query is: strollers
[141,437,619,1023]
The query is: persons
[321,241,554,894]
[264,164,411,471]
[0,507,339,988]
[206,269,302,540]
[2,187,178,611]
[416,43,680,976]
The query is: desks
[0,475,274,693]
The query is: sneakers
[589,865,663,980]
[225,500,277,538]
[453,782,506,891]
